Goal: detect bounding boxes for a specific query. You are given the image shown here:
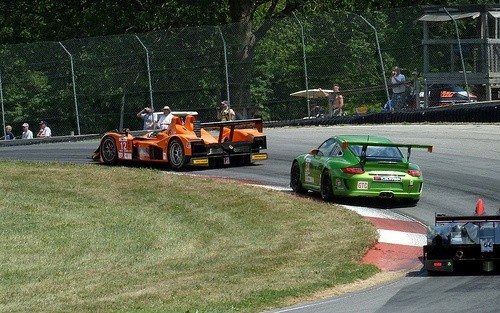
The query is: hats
[22,123,29,126]
[221,101,228,104]
[161,106,171,112]
[38,121,47,125]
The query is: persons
[0,126,15,140]
[156,106,175,130]
[21,123,33,139]
[388,67,406,111]
[137,106,157,130]
[319,83,343,116]
[401,71,421,110]
[36,120,51,137]
[215,101,235,122]
[382,95,397,112]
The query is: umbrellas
[290,89,334,117]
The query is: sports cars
[92,115,268,173]
[291,134,433,207]
[417,199,500,276]
